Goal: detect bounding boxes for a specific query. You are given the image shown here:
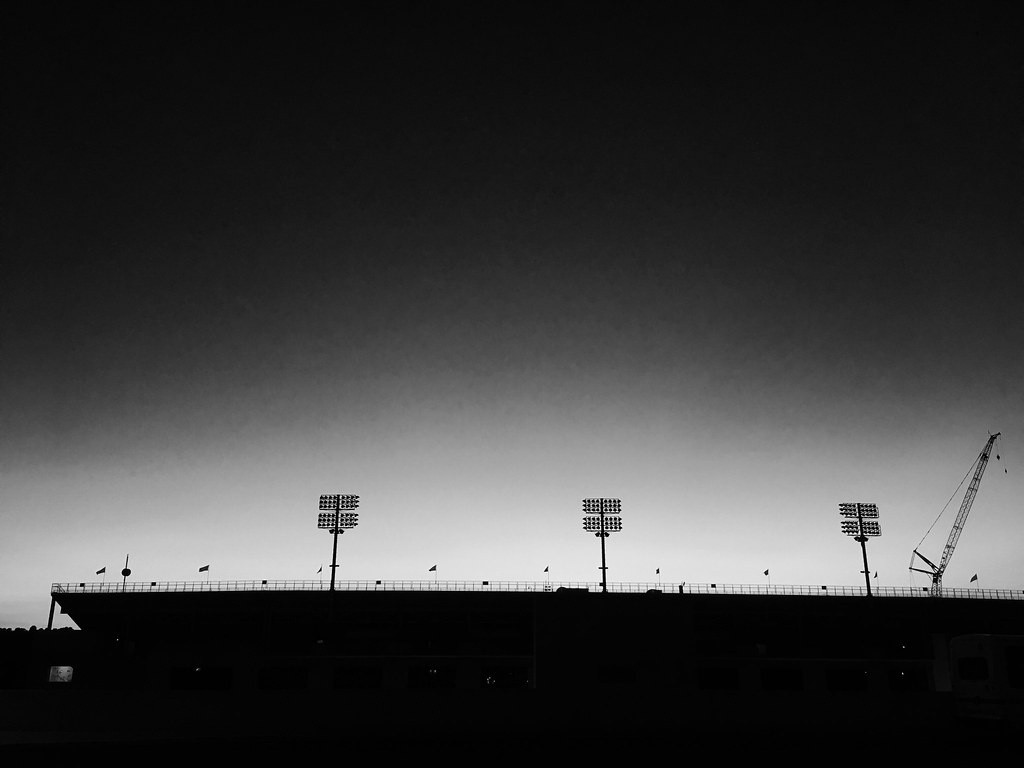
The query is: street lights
[839,500,882,596]
[582,497,623,592]
[315,492,360,588]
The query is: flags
[96,567,105,575]
[970,574,977,582]
[199,565,209,572]
[429,565,437,571]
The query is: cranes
[905,426,1006,593]
